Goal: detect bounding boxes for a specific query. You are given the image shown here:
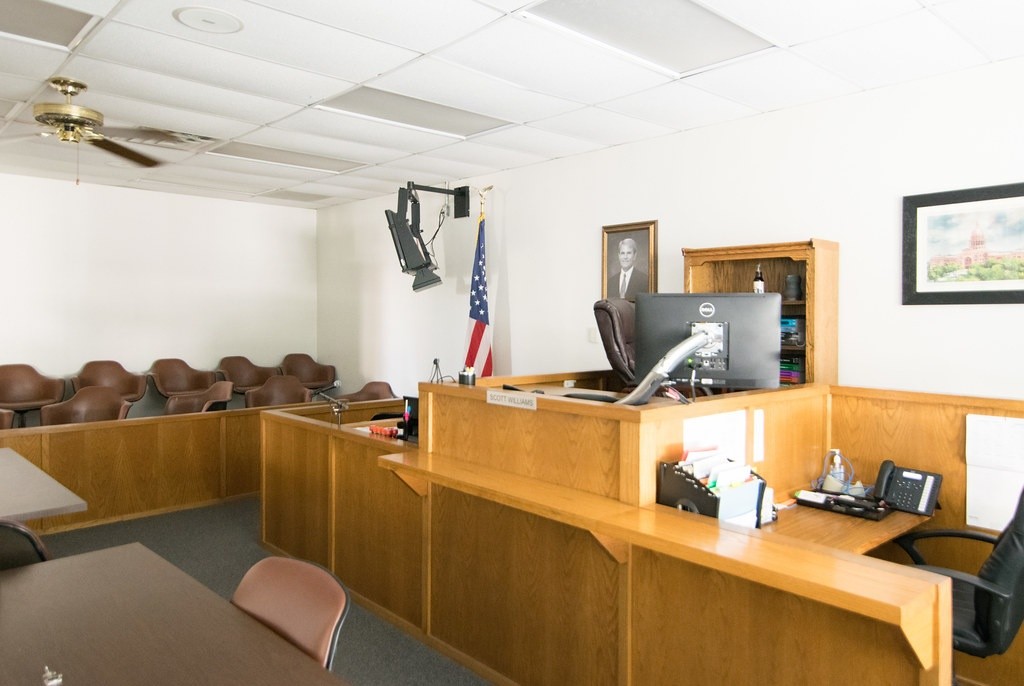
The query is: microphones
[311,380,342,397]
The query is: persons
[607,238,649,301]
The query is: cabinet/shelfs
[681,239,838,385]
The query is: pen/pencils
[460,363,475,373]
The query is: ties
[620,275,626,296]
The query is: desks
[757,502,937,555]
[0,541,353,686]
[0,447,88,562]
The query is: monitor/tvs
[635,293,781,389]
[386,210,417,276]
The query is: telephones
[871,460,943,516]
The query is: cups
[784,274,802,300]
[397,422,409,440]
[459,372,475,385]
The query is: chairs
[218,356,283,395]
[0,364,65,428]
[230,555,351,672]
[594,297,713,398]
[40,386,134,426]
[147,358,216,398]
[338,381,397,403]
[279,353,336,388]
[71,361,148,402]
[892,486,1024,658]
[244,375,311,408]
[163,380,234,415]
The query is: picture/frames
[601,220,658,301]
[902,183,1024,306]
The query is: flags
[463,212,493,378]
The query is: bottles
[753,270,764,293]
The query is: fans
[0,77,219,187]
